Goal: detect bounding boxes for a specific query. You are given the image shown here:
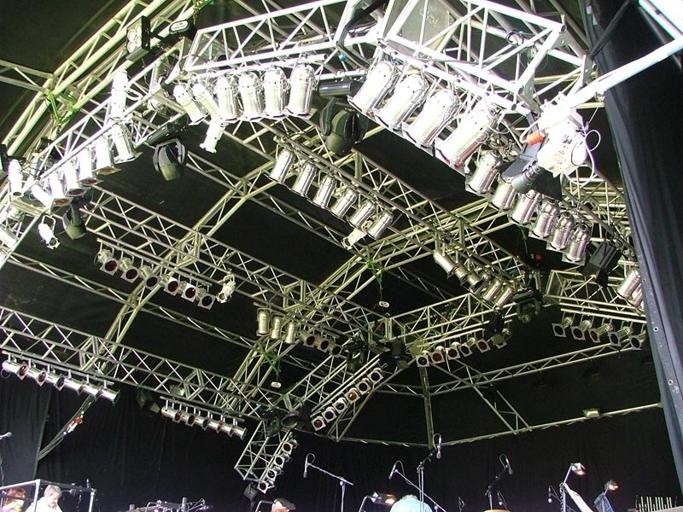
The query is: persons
[0,487,27,512]
[25,485,62,512]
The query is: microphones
[198,506,214,510]
[497,493,502,506]
[303,454,309,478]
[547,488,552,503]
[436,436,442,459]
[0,432,13,440]
[388,462,397,479]
[505,459,514,476]
